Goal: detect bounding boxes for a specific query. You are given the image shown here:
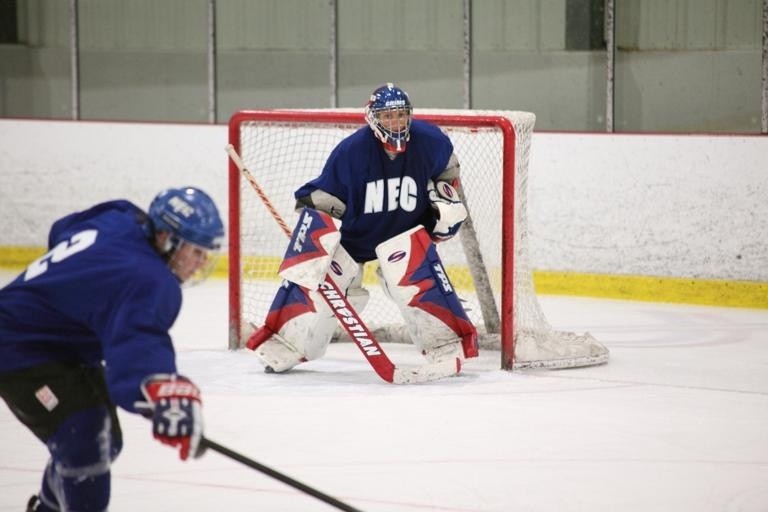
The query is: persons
[244,83,476,378]
[1,185,226,512]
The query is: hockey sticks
[226,144,462,385]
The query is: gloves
[141,373,204,460]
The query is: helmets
[149,187,224,250]
[369,84,412,110]
